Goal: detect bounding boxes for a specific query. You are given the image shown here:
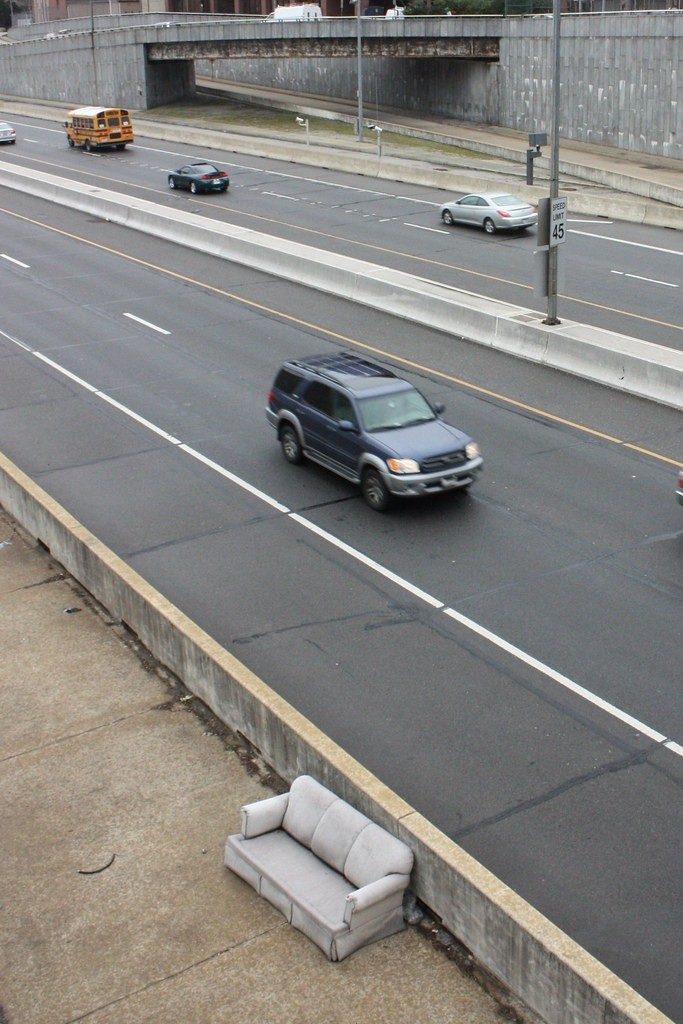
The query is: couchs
[222,775,414,963]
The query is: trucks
[260,4,323,21]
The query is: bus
[63,105,134,152]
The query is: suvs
[264,349,484,512]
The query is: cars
[167,162,230,195]
[438,190,538,236]
[0,123,16,145]
